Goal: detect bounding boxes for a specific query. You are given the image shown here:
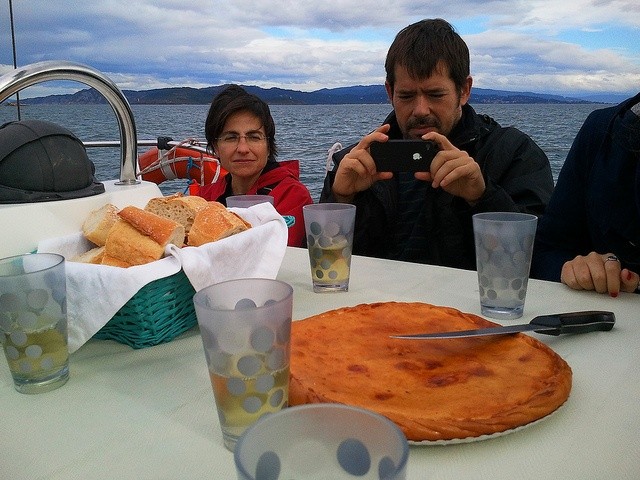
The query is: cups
[234,403,411,479]
[0,253,70,395]
[304,203,356,293]
[473,212,538,320]
[193,277,293,453]
[226,195,274,208]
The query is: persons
[192,84,313,248]
[319,18,554,279]
[529,92,640,297]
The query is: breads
[76,246,105,263]
[187,201,251,245]
[230,212,252,230]
[80,203,122,245]
[145,193,207,233]
[102,205,186,268]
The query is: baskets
[12,214,295,350]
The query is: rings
[604,255,621,265]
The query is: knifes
[388,311,616,339]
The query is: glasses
[215,132,269,141]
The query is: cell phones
[370,138,436,172]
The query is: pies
[289,301,573,442]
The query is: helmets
[0,120,105,204]
